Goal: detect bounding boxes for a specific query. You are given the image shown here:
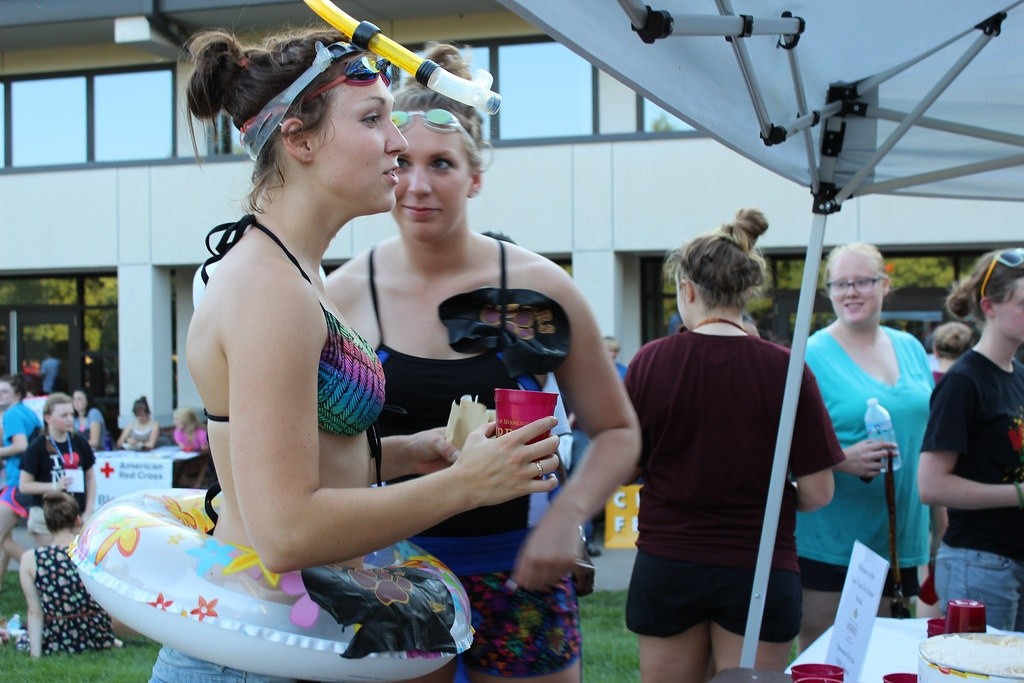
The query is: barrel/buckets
[917,633,1024,683]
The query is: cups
[791,664,844,683]
[494,389,559,480]
[883,673,917,683]
[927,599,986,638]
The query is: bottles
[864,399,902,472]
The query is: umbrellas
[494,0,1024,215]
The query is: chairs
[154,433,173,450]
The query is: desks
[93,445,207,512]
[783,616,1001,683]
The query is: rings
[534,460,544,476]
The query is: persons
[877,315,989,361]
[797,242,949,664]
[0,349,217,658]
[620,210,845,683]
[600,336,629,380]
[915,323,978,620]
[918,248,1024,632]
[147,29,559,683]
[326,45,640,683]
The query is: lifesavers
[67,481,476,683]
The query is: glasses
[242,55,393,137]
[387,108,480,151]
[980,246,1024,299]
[821,277,888,293]
